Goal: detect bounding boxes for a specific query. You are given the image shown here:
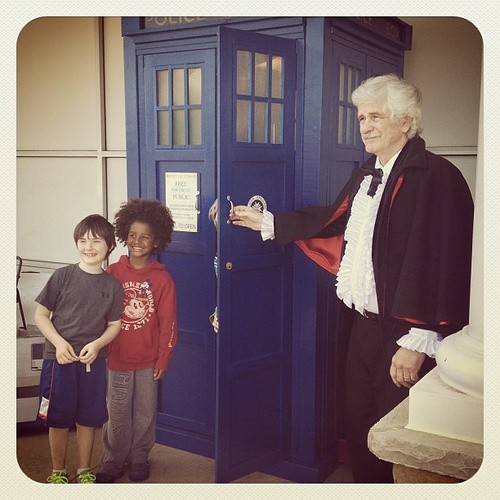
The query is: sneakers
[76,468,95,484]
[46,468,70,484]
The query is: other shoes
[95,472,114,484]
[129,462,148,480]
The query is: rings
[403,377,410,378]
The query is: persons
[96,197,179,483]
[229,71,474,484]
[33,214,125,484]
